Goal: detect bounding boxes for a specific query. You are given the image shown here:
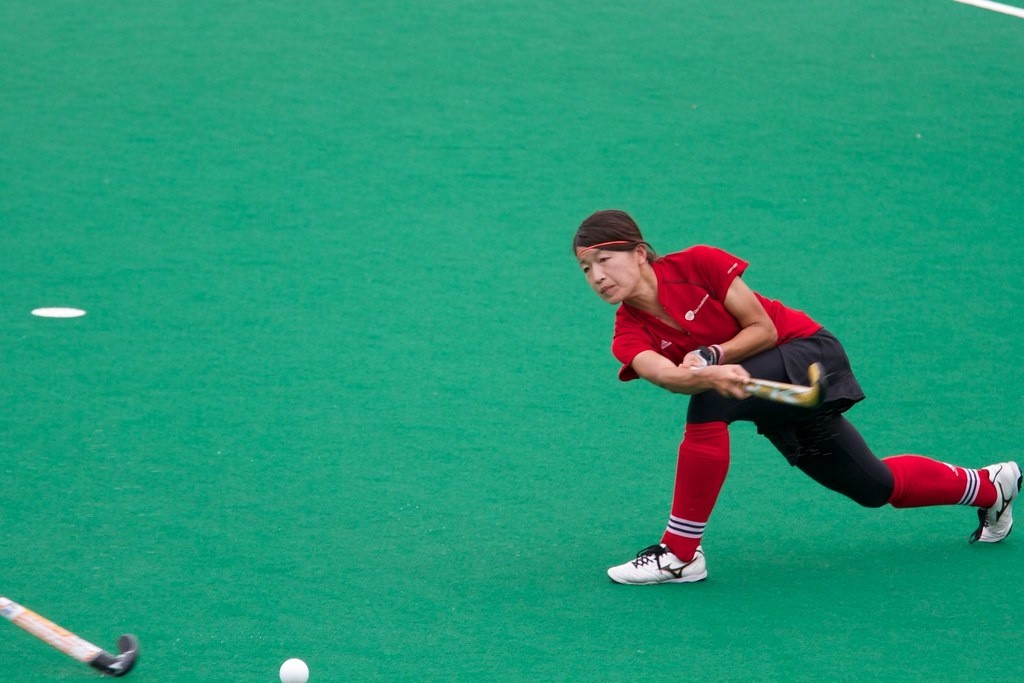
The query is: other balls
[277,657,311,683]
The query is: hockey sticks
[673,359,826,410]
[1,597,141,679]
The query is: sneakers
[968,461,1024,544]
[607,543,708,585]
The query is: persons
[575,210,1023,586]
